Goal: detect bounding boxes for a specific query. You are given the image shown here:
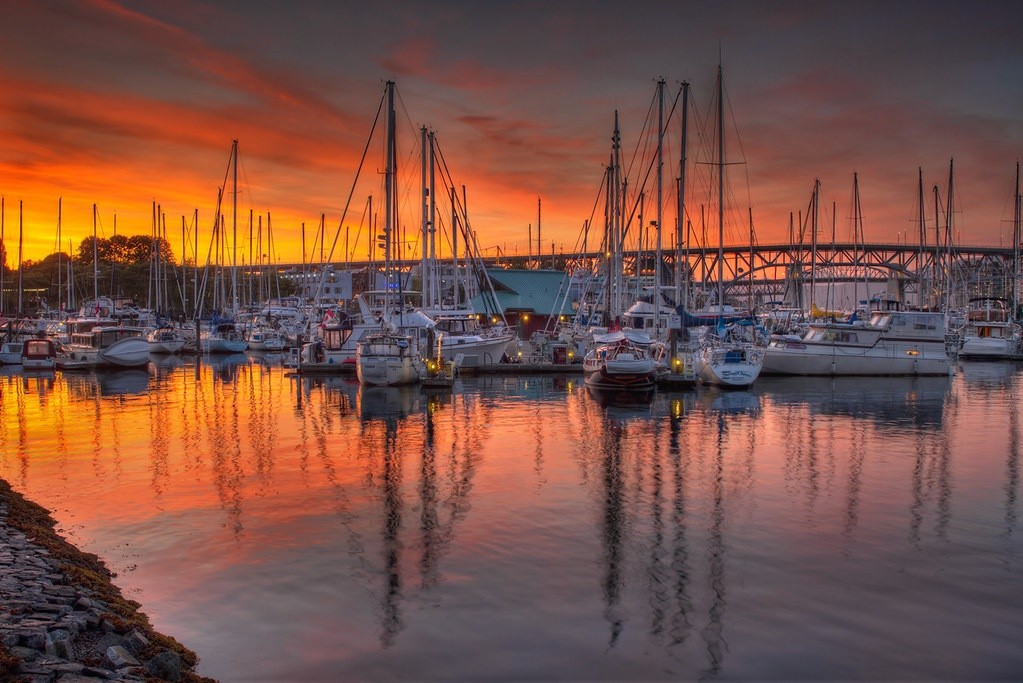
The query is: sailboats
[1,45,1023,396]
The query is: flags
[320,309,335,327]
[118,317,125,335]
[94,301,101,327]
